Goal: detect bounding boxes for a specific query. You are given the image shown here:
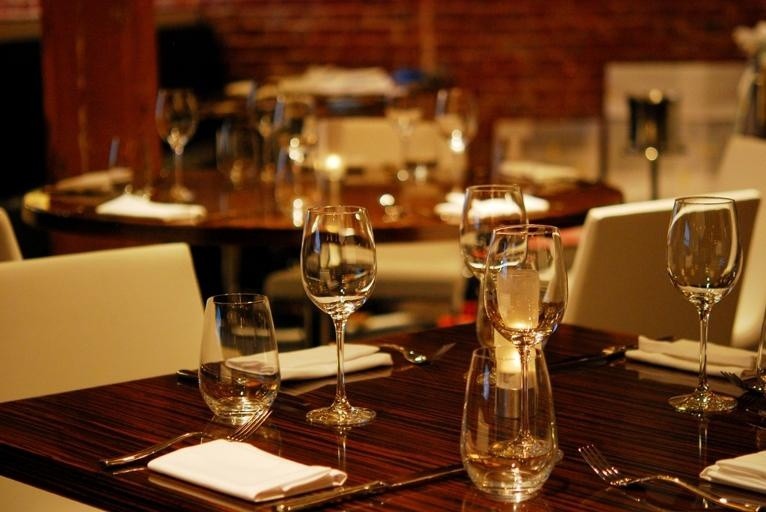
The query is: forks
[578,443,765,511]
[98,408,274,473]
[382,341,456,366]
[721,369,765,396]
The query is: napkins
[699,448,764,494]
[225,341,393,381]
[626,331,764,381]
[147,438,346,503]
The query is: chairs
[1,242,229,511]
[3,61,764,202]
[558,186,765,347]
[0,211,29,261]
[264,108,473,347]
[703,133,765,351]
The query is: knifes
[549,336,673,372]
[273,461,465,511]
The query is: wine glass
[153,88,202,204]
[434,88,479,217]
[301,203,379,427]
[461,184,529,280]
[484,225,569,459]
[663,196,743,413]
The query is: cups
[459,345,558,503]
[197,292,281,426]
